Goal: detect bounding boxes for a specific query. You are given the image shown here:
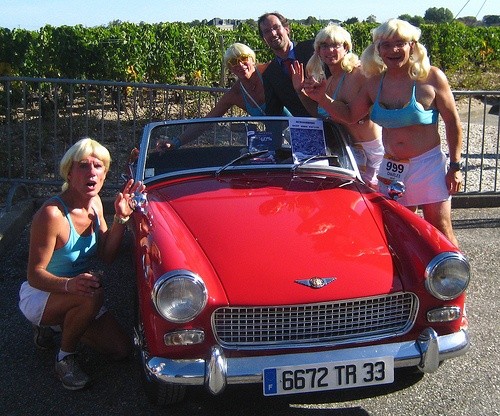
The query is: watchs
[450,162,462,171]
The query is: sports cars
[120,116,472,410]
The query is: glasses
[227,56,250,65]
[319,42,343,49]
[380,41,406,47]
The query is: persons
[261,12,330,149]
[19,137,147,390]
[304,19,468,331]
[290,25,385,185]
[154,42,271,150]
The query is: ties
[281,60,293,83]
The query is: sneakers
[460,304,468,331]
[54,354,88,390]
[33,322,55,350]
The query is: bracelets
[114,214,130,225]
[170,137,182,148]
[65,278,70,294]
